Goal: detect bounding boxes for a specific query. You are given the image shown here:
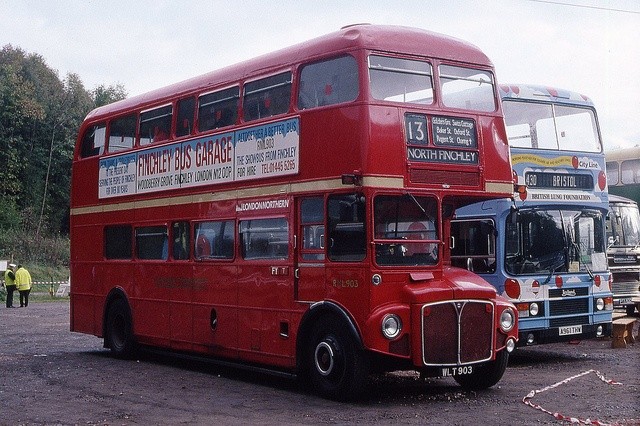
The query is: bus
[602,145,640,204]
[69,22,526,392]
[609,193,639,334]
[163,84,618,347]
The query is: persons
[5,264,16,308]
[16,265,32,307]
